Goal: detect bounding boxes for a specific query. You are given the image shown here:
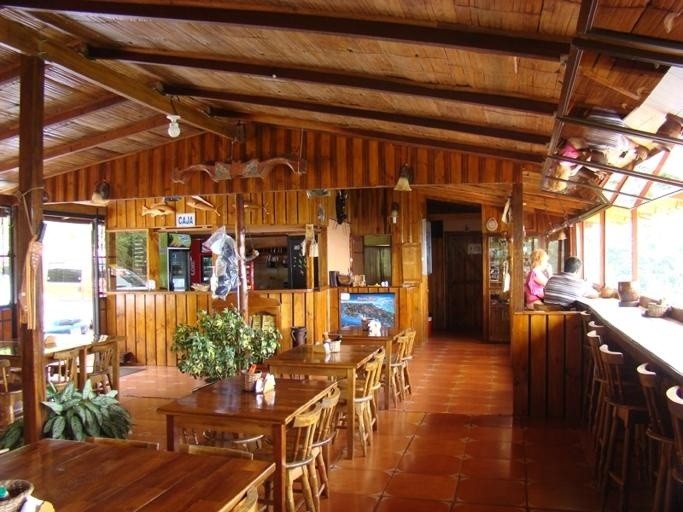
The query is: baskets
[330,340,341,352]
[240,369,263,392]
[648,303,670,317]
[194,284,209,291]
[601,288,612,298]
[0,480,35,512]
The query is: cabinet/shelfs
[482,233,550,342]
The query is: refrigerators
[189,238,213,291]
[158,233,192,291]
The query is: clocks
[485,216,498,231]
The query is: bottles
[361,313,369,332]
[322,332,331,342]
[1,486,13,502]
[331,335,343,342]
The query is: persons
[524,248,553,310]
[546,107,647,194]
[543,256,599,308]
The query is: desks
[0,334,127,425]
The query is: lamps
[92,180,110,204]
[393,163,413,192]
[557,230,567,240]
[389,201,400,225]
[166,116,182,137]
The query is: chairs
[84,436,159,452]
[233,324,416,512]
[86,341,116,397]
[0,360,23,425]
[51,348,80,395]
[579,297,683,512]
